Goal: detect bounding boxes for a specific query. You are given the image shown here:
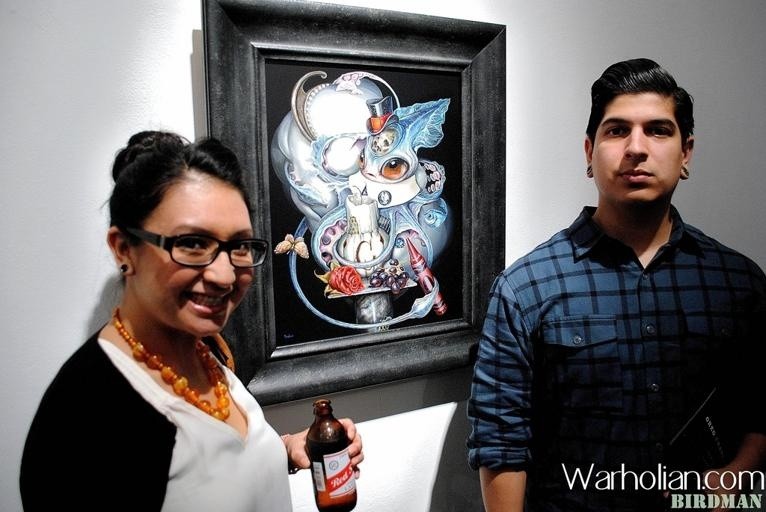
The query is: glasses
[126,228,269,268]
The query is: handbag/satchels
[668,387,744,476]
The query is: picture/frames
[202,0,507,408]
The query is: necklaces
[108,306,232,423]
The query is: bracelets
[278,433,301,477]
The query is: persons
[14,126,366,511]
[460,52,765,512]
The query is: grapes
[370,259,411,295]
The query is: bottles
[305,400,358,511]
